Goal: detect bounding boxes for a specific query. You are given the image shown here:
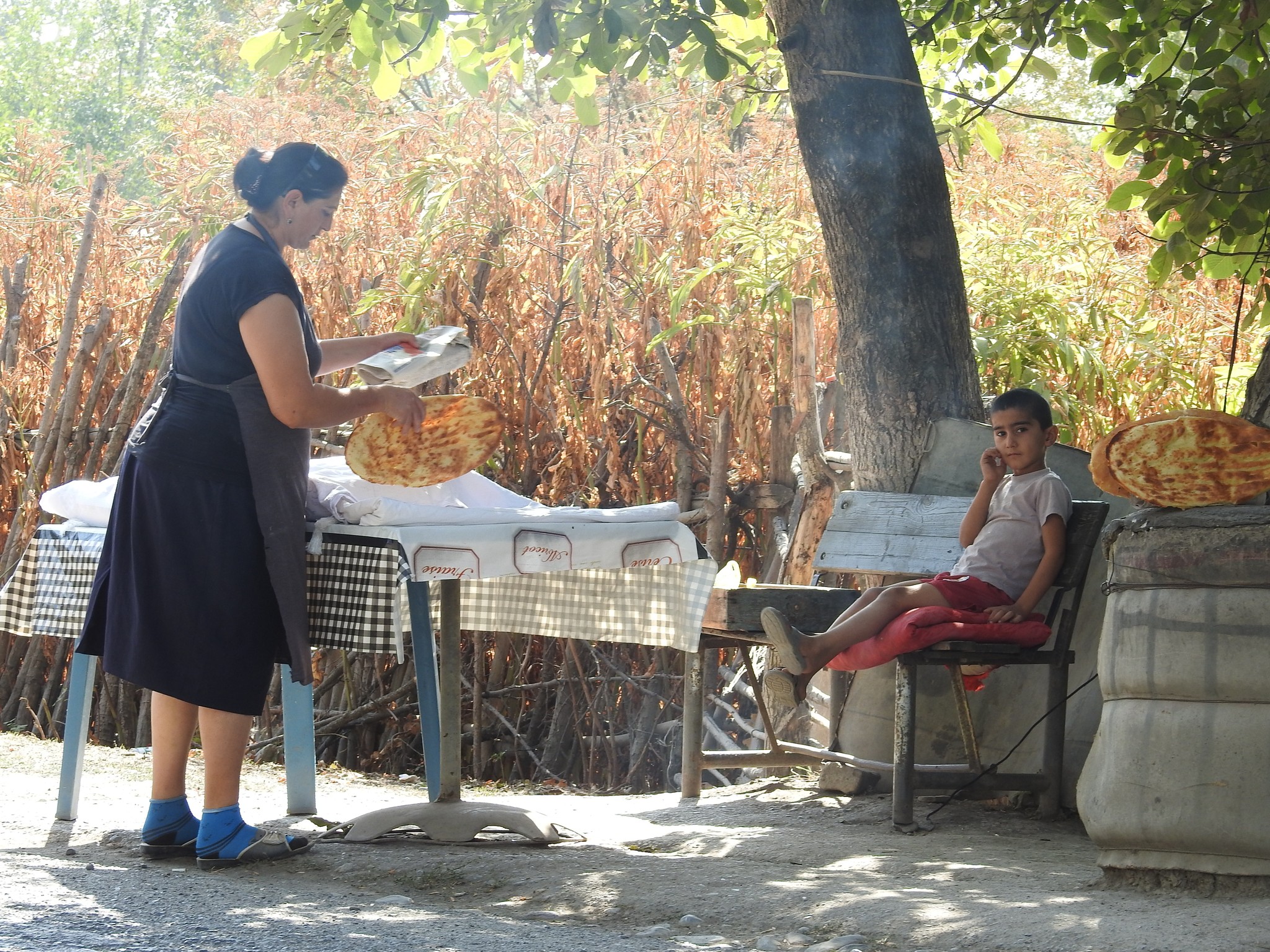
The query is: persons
[760,388,1073,706]
[73,142,429,874]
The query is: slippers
[139,835,197,858]
[197,830,316,870]
[764,670,800,707]
[761,607,808,676]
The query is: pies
[1088,408,1270,510]
[345,394,505,488]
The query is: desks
[0,520,720,827]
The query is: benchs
[681,489,1114,833]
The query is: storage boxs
[702,583,862,632]
